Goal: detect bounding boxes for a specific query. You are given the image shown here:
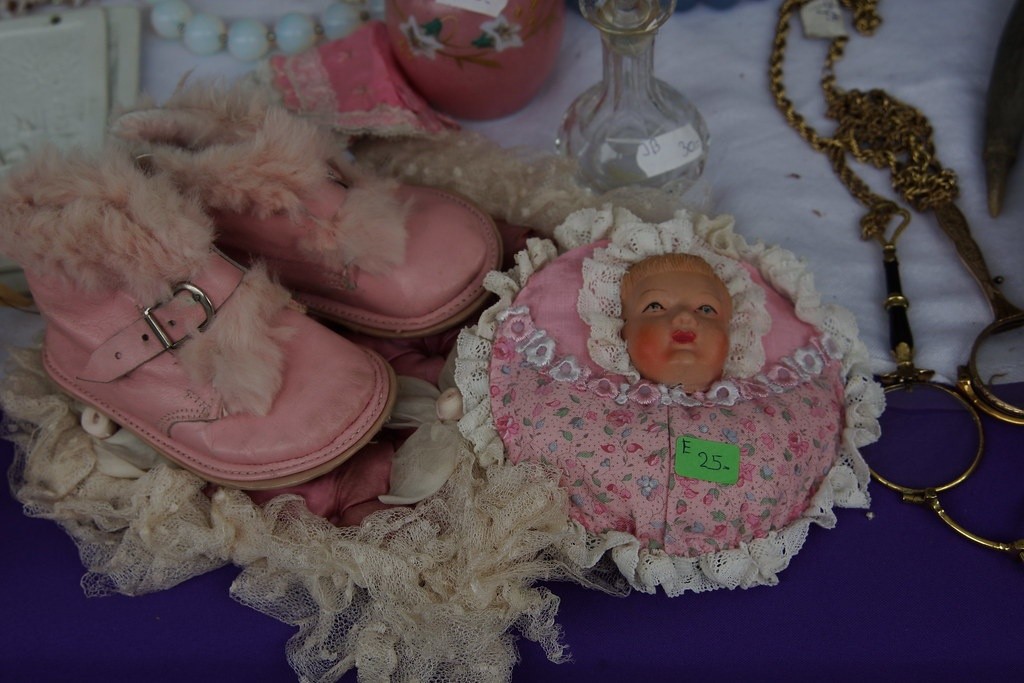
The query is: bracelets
[147,0,385,61]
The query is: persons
[583,217,769,393]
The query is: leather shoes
[0,142,397,489]
[108,89,503,338]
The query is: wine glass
[553,0,710,203]
